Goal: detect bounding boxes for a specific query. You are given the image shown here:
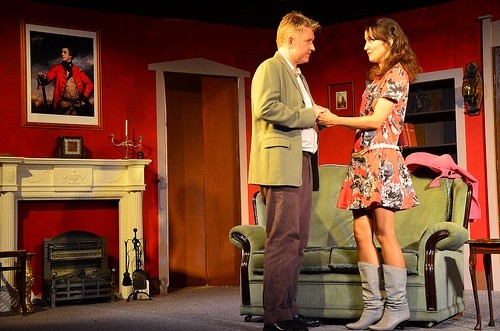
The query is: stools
[464,239,500,331]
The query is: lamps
[462,62,483,115]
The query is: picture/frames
[18,17,103,130]
[57,136,84,158]
[327,81,354,116]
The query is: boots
[368,264,411,330]
[346,263,385,330]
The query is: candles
[126,120,127,137]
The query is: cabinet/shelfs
[395,66,467,176]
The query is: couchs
[229,164,474,327]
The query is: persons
[247,12,329,331]
[318,18,421,331]
[37,44,94,115]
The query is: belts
[61,96,80,104]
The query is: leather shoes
[293,314,320,327]
[264,317,309,331]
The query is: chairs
[0,250,27,316]
